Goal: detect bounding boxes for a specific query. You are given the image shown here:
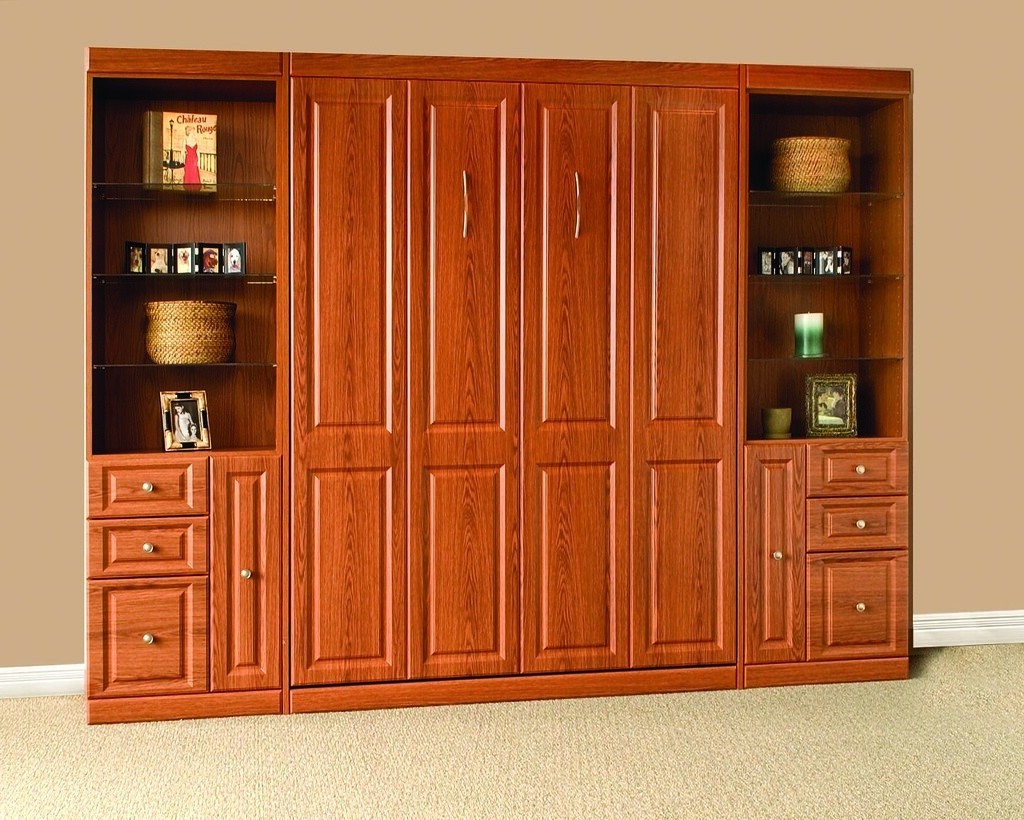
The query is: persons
[179,125,204,192]
[172,401,201,442]
[817,387,848,427]
[761,249,850,276]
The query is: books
[140,109,218,192]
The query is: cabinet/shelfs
[83,43,917,729]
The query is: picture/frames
[775,246,798,276]
[816,246,838,276]
[804,372,859,438]
[801,246,814,276]
[147,243,172,274]
[198,243,223,274]
[174,242,195,275]
[756,246,774,276]
[840,245,853,275]
[160,390,213,452]
[224,242,246,275]
[124,240,146,275]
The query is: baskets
[771,136,851,193]
[144,300,238,364]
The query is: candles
[793,312,828,359]
